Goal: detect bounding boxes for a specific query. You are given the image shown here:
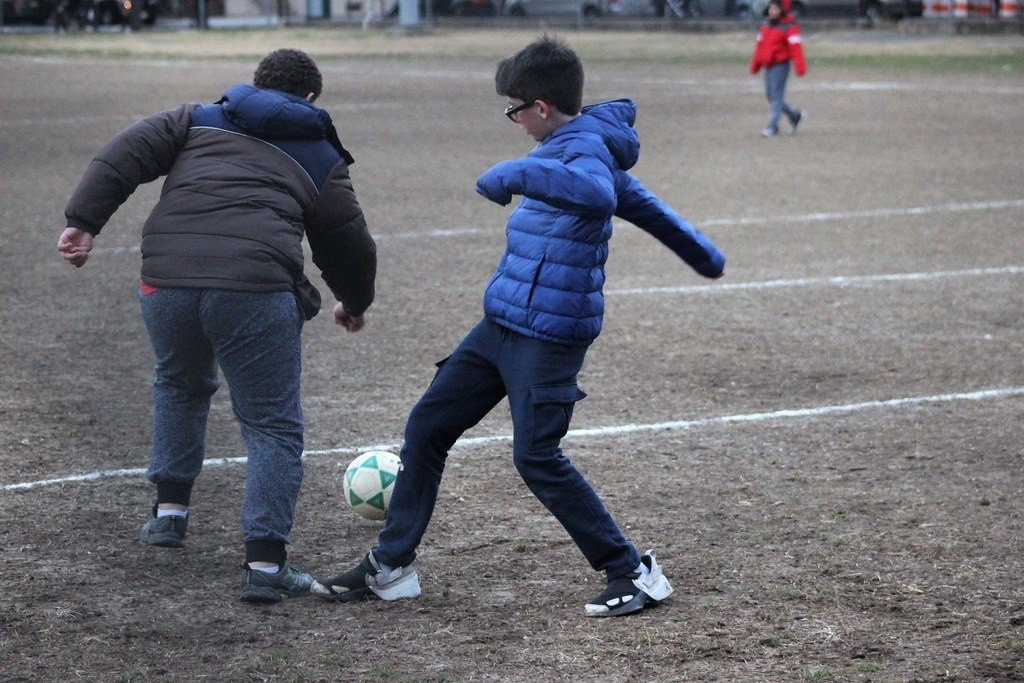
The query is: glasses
[505,100,531,123]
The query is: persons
[749,0,807,137]
[56,48,377,600]
[312,41,727,617]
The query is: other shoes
[761,127,778,136]
[787,112,807,136]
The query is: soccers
[343,450,401,521]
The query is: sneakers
[239,562,313,602]
[140,504,189,548]
[310,545,421,601]
[583,549,675,617]
